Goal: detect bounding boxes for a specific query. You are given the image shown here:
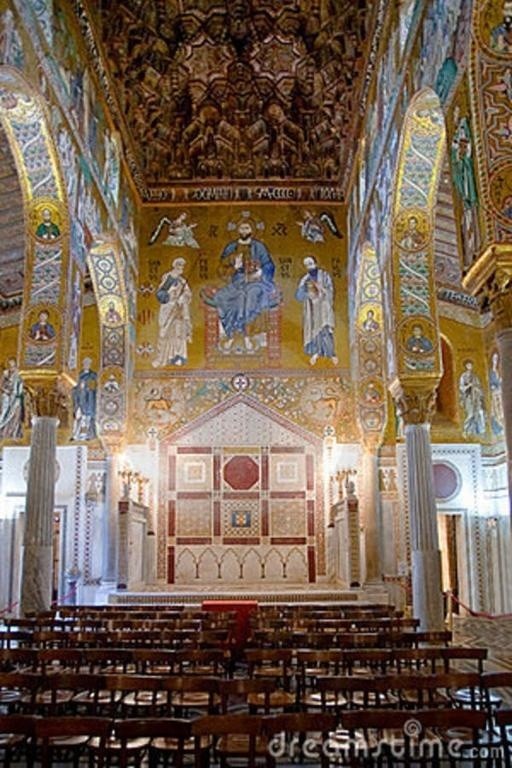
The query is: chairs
[0,605,512,768]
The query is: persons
[105,301,122,325]
[25,309,57,341]
[36,209,61,241]
[200,212,282,356]
[294,208,326,244]
[407,324,432,353]
[400,216,425,250]
[151,256,194,367]
[362,381,381,403]
[1,357,27,442]
[459,359,487,440]
[69,355,100,444]
[162,208,200,249]
[104,374,118,393]
[488,352,503,437]
[362,309,379,333]
[295,254,342,367]
